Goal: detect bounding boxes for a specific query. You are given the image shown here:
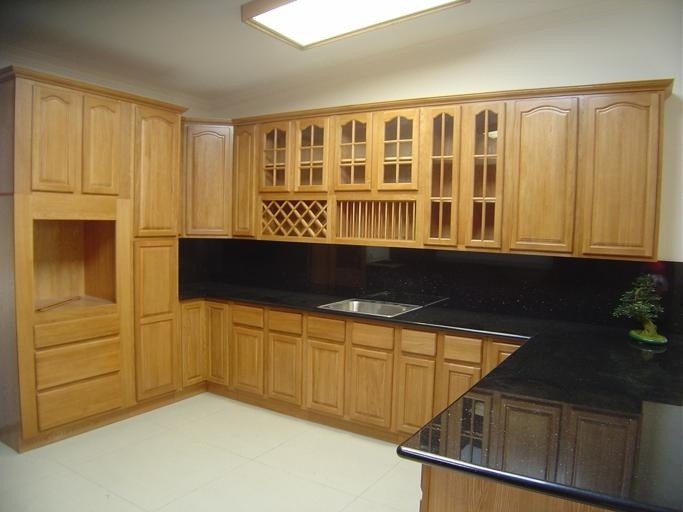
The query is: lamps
[237,0,477,52]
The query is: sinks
[318,297,423,318]
[365,289,447,307]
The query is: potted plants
[615,271,670,346]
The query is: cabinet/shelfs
[232,106,334,244]
[417,90,504,254]
[415,397,492,466]
[333,93,417,250]
[481,334,524,376]
[182,112,236,241]
[134,95,191,417]
[503,77,675,263]
[204,299,230,402]
[0,62,135,455]
[229,302,301,421]
[492,395,638,500]
[394,323,485,448]
[302,312,393,441]
[182,303,204,399]
